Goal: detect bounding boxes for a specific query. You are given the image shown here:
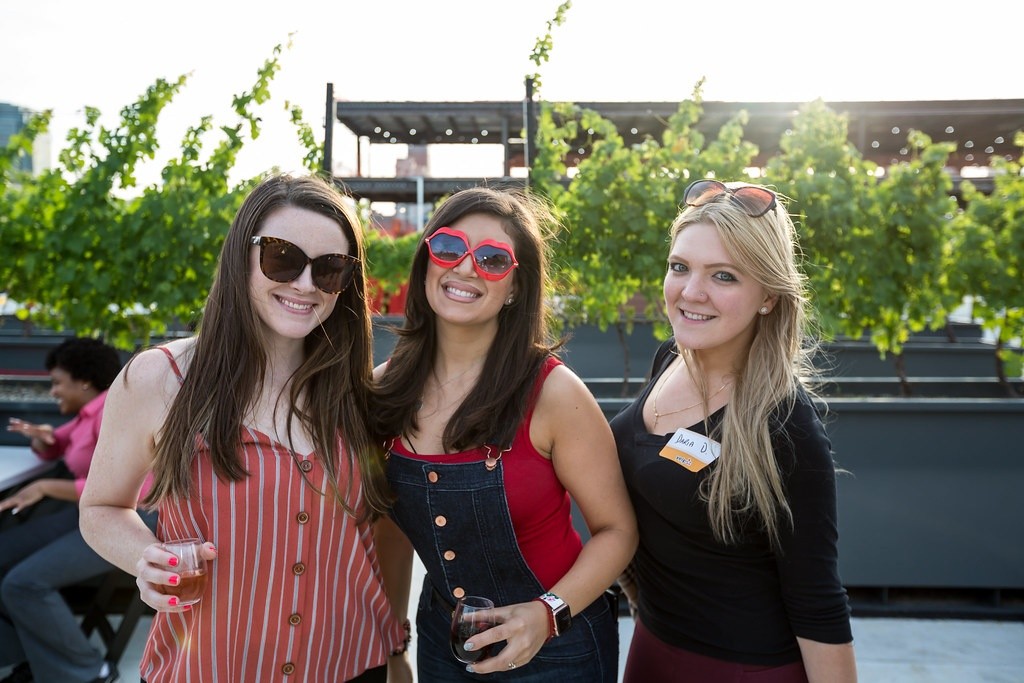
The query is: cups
[159,538,208,605]
[450,596,495,664]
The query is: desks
[0,444,63,497]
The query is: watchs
[539,592,572,636]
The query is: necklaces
[653,357,730,427]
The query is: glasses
[425,226,519,281]
[677,180,778,219]
[251,236,362,294]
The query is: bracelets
[389,620,410,656]
[533,598,554,643]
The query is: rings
[508,662,516,669]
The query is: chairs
[54,534,160,671]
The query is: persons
[608,182,858,683]
[81,175,407,683]
[371,187,640,683]
[0,335,158,683]
